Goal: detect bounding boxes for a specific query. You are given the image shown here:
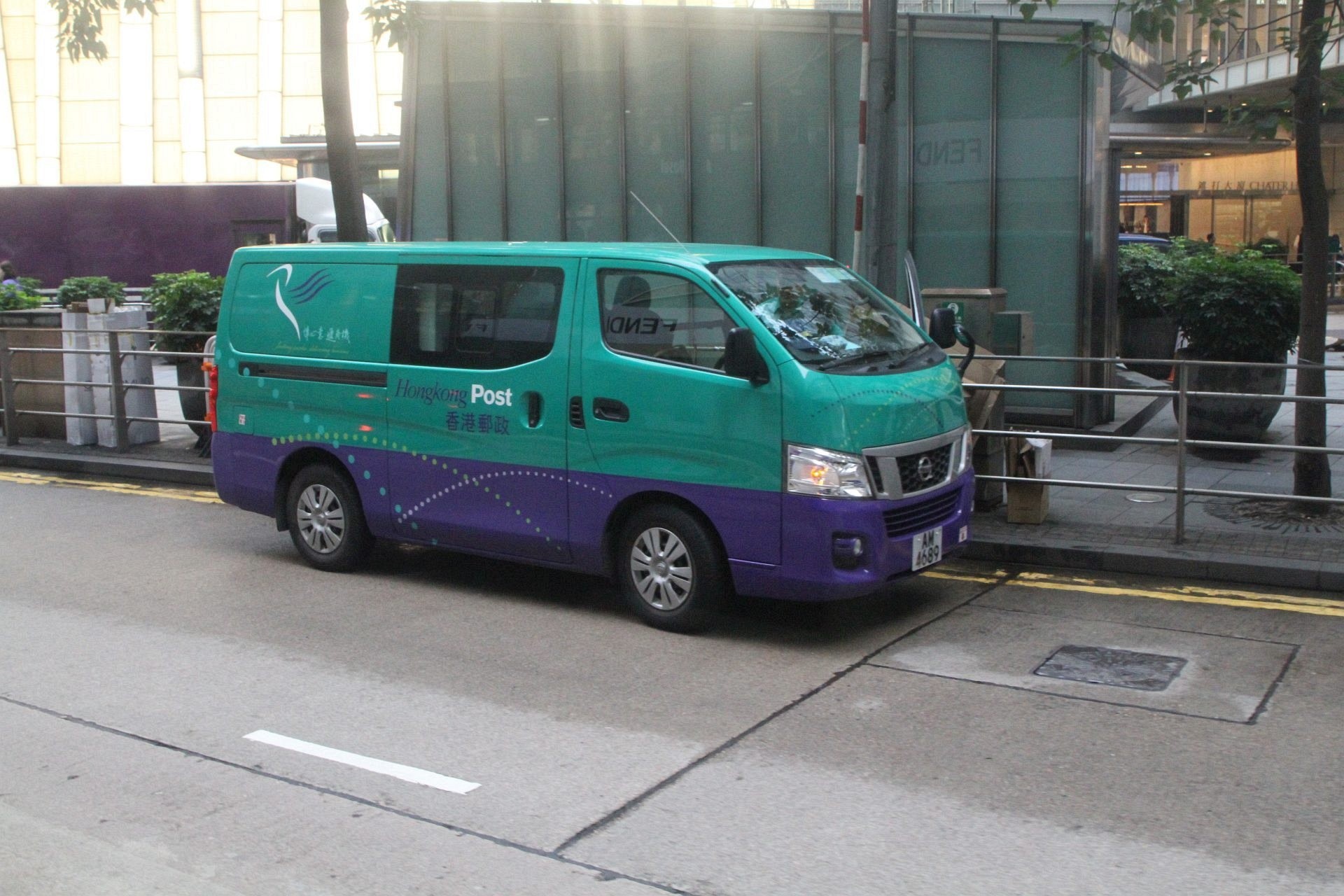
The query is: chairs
[608,273,672,354]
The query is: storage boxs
[1003,424,1053,524]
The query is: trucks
[0,175,397,351]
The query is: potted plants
[1116,238,1183,381]
[1159,243,1302,451]
[146,267,227,441]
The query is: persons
[0,260,24,300]
[1207,232,1215,247]
[1141,215,1150,233]
[752,265,844,341]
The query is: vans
[209,239,977,636]
